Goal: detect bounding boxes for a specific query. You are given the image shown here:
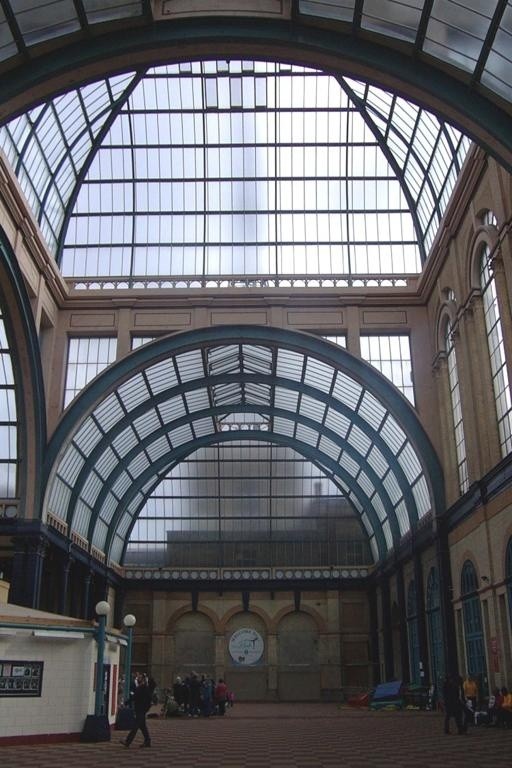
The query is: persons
[119,673,152,748]
[129,671,235,720]
[461,675,511,730]
[438,665,467,735]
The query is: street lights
[123,614,135,708]
[95,601,110,717]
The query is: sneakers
[139,740,152,747]
[119,739,129,747]
